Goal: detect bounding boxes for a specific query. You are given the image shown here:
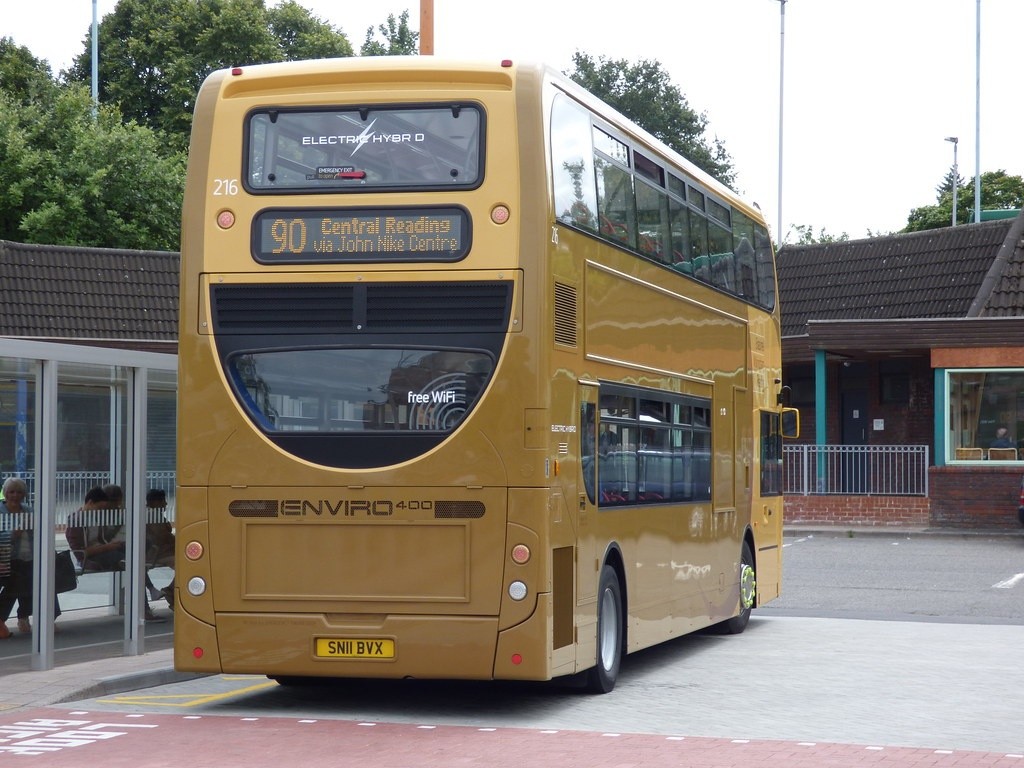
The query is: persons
[985,428,1022,460]
[146,489,174,611]
[65,484,169,624]
[0,476,34,640]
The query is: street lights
[945,136,958,227]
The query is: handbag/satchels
[53,550,77,594]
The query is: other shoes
[0,620,9,638]
[17,616,31,632]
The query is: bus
[170,52,801,694]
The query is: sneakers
[146,605,168,623]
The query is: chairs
[988,448,1019,461]
[954,448,983,460]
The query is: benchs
[71,560,170,615]
